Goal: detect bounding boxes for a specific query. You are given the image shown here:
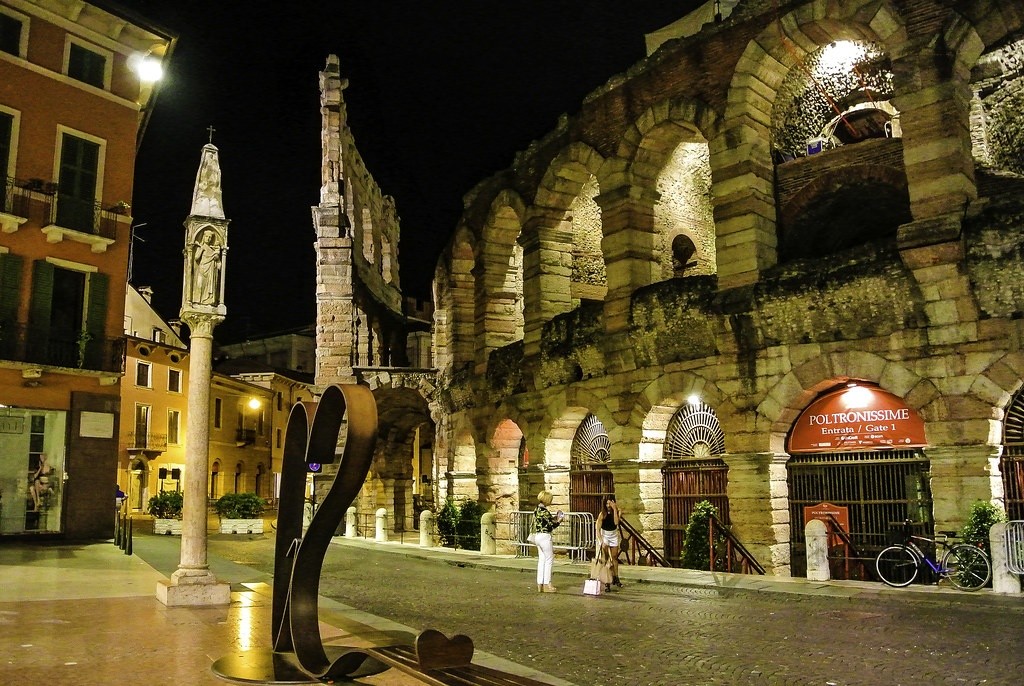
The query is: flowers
[114,201,131,209]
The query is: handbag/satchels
[527,533,536,544]
[590,546,613,584]
[582,578,601,595]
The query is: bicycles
[876,518,992,592]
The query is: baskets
[885,531,904,543]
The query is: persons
[533,491,563,593]
[30,453,56,512]
[595,494,623,592]
[115,484,129,527]
[191,229,221,304]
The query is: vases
[114,207,125,215]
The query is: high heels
[537,584,543,592]
[605,583,611,592]
[613,575,624,587]
[544,584,556,592]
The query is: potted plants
[27,177,45,192]
[215,493,266,534]
[46,181,59,195]
[147,490,183,535]
